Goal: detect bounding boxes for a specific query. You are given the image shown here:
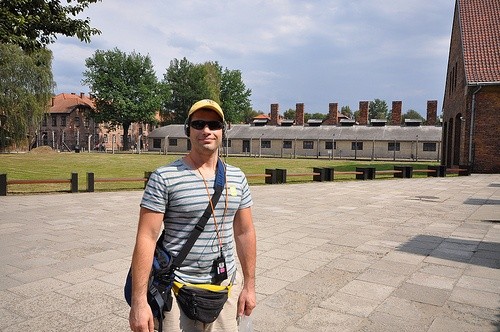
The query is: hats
[188,99,225,122]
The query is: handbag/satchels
[124,229,176,332]
[172,281,233,323]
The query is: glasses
[188,119,225,130]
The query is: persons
[129,99,256,332]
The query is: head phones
[184,116,226,138]
